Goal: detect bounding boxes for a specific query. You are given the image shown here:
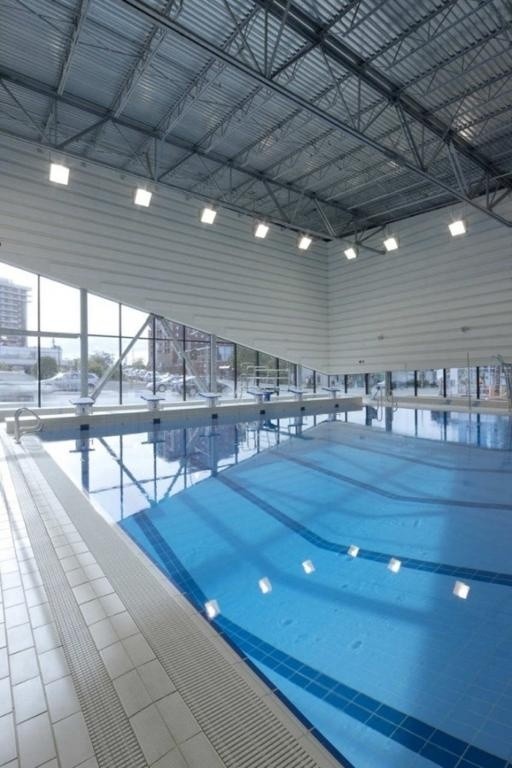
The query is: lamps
[200,206,217,225]
[134,187,152,207]
[254,202,473,261]
[48,162,69,187]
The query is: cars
[373,378,438,388]
[122,367,232,397]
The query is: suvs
[38,371,99,394]
